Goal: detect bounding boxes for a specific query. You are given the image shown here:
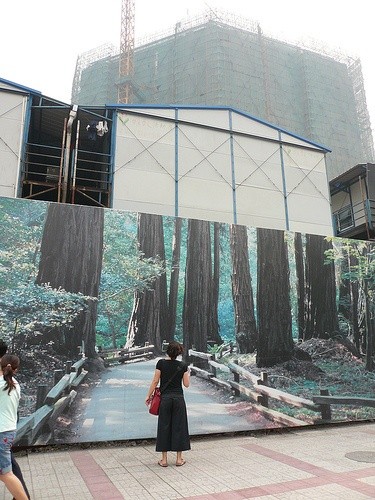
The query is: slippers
[175,459,185,466]
[158,460,167,466]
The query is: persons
[145,341,190,466]
[0,339,30,500]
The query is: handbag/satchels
[149,388,160,415]
